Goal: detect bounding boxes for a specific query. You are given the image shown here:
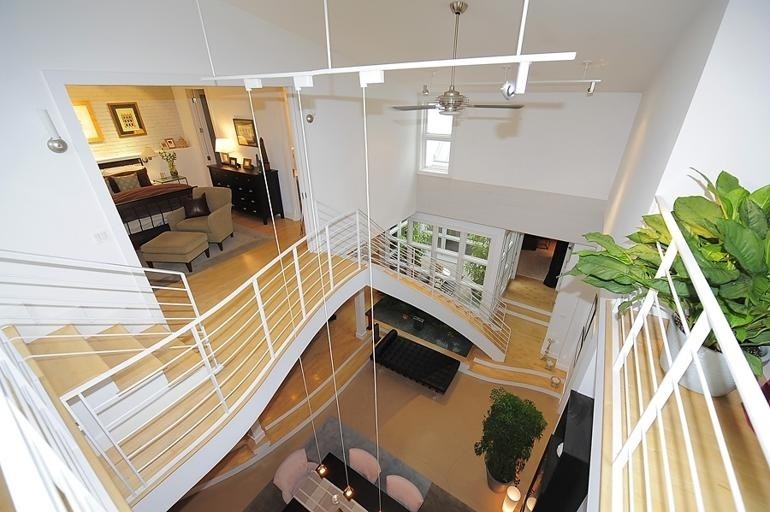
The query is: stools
[140,231,210,273]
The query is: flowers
[159,150,177,168]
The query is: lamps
[502,486,522,512]
[439,109,462,116]
[500,66,517,101]
[422,85,429,95]
[214,137,235,153]
[245,68,362,503]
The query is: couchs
[369,328,462,399]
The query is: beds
[95,155,198,251]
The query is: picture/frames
[71,99,106,144]
[233,118,258,147]
[107,102,147,138]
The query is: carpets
[128,223,275,290]
[516,247,552,281]
[366,296,474,357]
[242,418,432,512]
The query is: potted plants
[471,383,549,492]
[552,161,770,398]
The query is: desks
[154,175,189,187]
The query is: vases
[169,168,178,177]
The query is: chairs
[270,447,424,512]
[166,186,233,251]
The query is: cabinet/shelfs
[206,164,284,225]
[520,390,593,512]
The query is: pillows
[100,164,153,194]
[180,193,213,220]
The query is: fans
[390,0,524,110]
